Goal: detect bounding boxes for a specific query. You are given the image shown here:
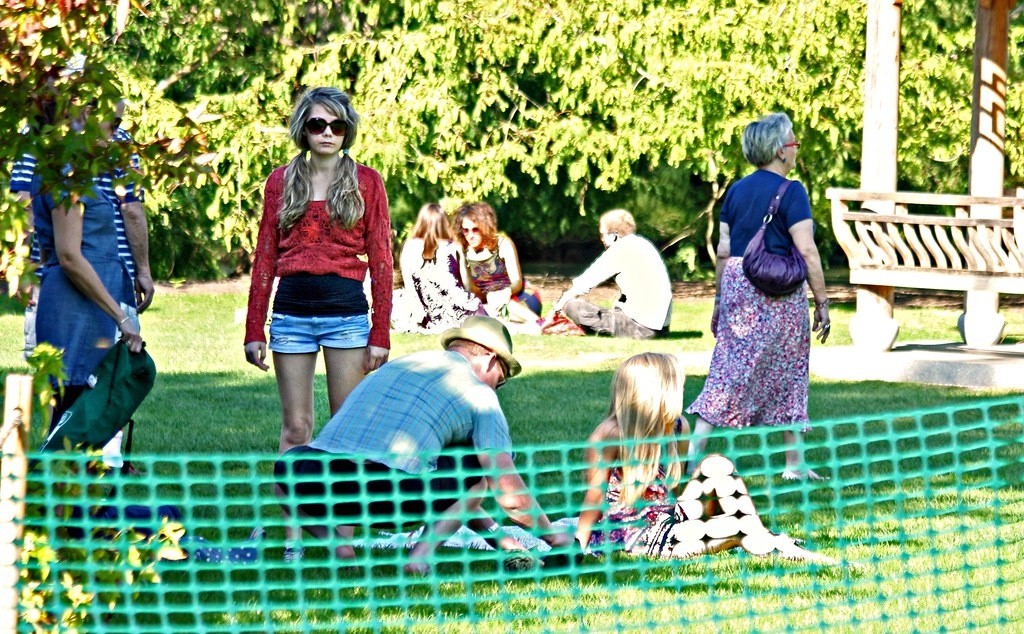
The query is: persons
[684,115,830,481]
[551,209,673,340]
[400,203,488,328]
[270,316,575,576]
[244,88,394,567]
[453,201,542,325]
[10,55,155,534]
[571,353,802,560]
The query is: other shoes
[283,538,307,562]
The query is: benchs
[825,187,1024,349]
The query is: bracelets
[120,317,129,323]
[814,299,829,307]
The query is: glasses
[461,226,481,233]
[303,117,350,136]
[495,356,509,391]
[782,140,801,148]
[110,117,122,131]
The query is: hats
[441,315,522,380]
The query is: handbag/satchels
[742,178,807,296]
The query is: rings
[825,325,830,328]
